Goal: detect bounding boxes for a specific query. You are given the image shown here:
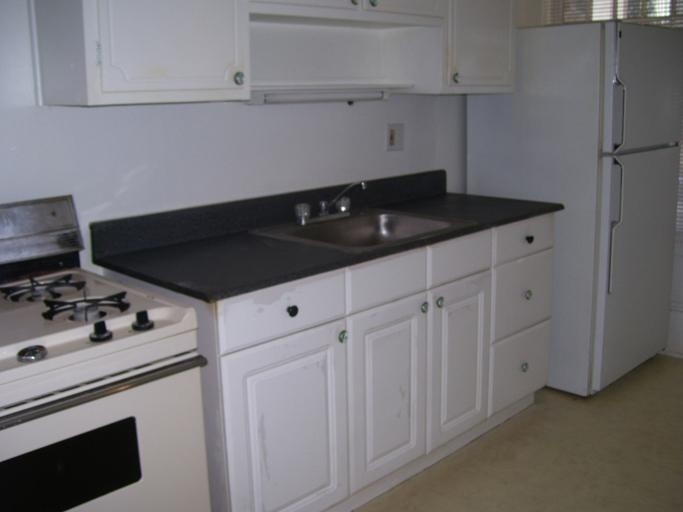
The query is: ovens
[0,346,215,512]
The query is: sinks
[284,212,450,249]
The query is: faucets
[319,178,366,217]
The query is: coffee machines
[462,22,682,405]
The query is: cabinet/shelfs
[448,0,514,95]
[29,0,252,106]
[494,203,563,427]
[252,2,446,100]
[352,227,493,511]
[116,264,354,512]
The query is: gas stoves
[0,269,198,415]
